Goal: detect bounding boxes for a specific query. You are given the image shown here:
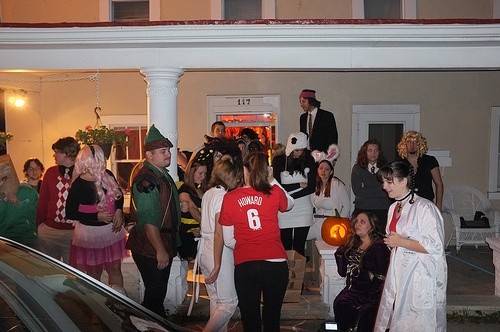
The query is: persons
[197,160,241,332]
[19,158,44,194]
[174,120,262,264]
[349,139,394,229]
[332,210,391,332]
[397,131,444,214]
[305,160,350,241]
[299,90,338,155]
[0,186,42,247]
[65,144,130,285]
[127,125,190,316]
[374,159,447,332]
[218,153,295,332]
[272,132,317,258]
[35,138,82,266]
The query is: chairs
[444,185,500,255]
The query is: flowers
[75,125,132,144]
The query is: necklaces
[396,192,411,213]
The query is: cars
[0,236,200,332]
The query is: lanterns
[320,217,353,246]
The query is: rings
[195,235,196,236]
[187,207,189,209]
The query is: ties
[63,168,70,179]
[371,166,375,175]
[309,114,313,139]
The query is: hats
[282,132,307,177]
[143,124,173,152]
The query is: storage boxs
[0,153,20,194]
[282,250,307,303]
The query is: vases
[88,144,113,159]
